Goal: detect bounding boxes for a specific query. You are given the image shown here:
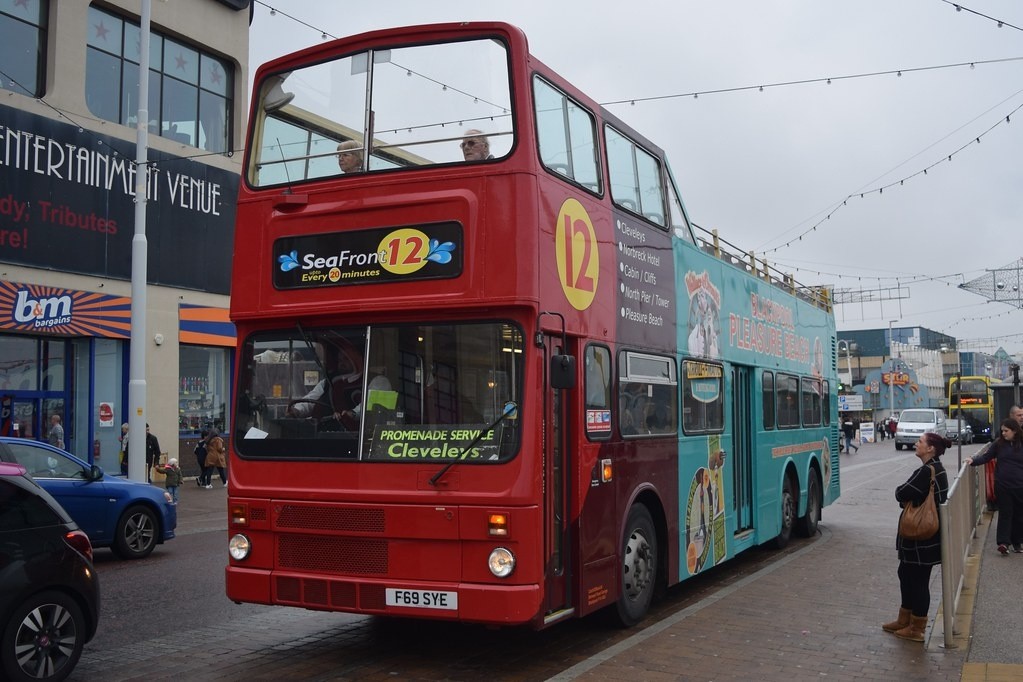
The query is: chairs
[766,408,821,429]
[618,392,674,434]
[547,162,828,310]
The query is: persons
[884,416,888,431]
[194,430,209,488]
[204,427,228,488]
[880,421,885,441]
[853,418,859,439]
[842,417,858,454]
[889,419,896,439]
[48,415,65,472]
[995,405,1023,439]
[962,417,1023,555]
[284,339,392,431]
[119,423,128,474]
[882,432,952,641]
[122,422,160,484]
[155,458,183,505]
[838,417,844,452]
[462,128,496,161]
[336,140,363,173]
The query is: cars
[1,436,178,560]
[946,418,975,445]
[0,462,103,682]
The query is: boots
[882,607,912,633]
[894,613,929,642]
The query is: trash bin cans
[150,451,169,483]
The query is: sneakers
[997,543,1010,554]
[1012,542,1023,552]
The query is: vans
[894,409,946,452]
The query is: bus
[948,376,1003,443]
[214,14,842,628]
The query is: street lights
[887,319,901,423]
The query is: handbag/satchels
[899,465,939,541]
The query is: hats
[167,458,177,465]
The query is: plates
[683,467,714,575]
[813,337,823,372]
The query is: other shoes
[224,480,228,487]
[206,484,212,488]
[201,483,206,487]
[196,477,201,486]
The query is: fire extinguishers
[94,440,100,459]
